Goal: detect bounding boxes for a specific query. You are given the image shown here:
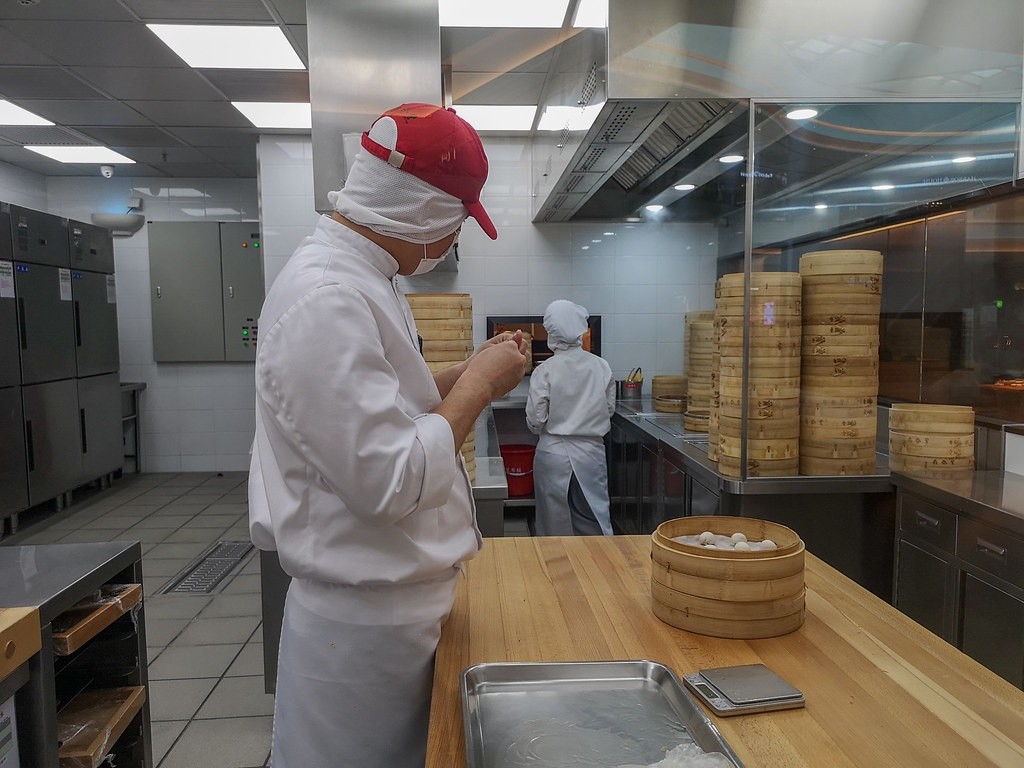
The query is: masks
[410,234,463,278]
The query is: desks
[425,535,1024,767]
[121,381,146,473]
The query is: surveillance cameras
[101,166,114,178]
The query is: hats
[358,100,502,243]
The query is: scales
[682,663,806,717]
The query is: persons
[525,300,615,537]
[257,105,527,767]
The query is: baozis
[735,542,750,550]
[731,533,747,545]
[699,531,715,545]
[761,540,777,550]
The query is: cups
[621,379,643,399]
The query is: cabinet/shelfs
[892,486,1024,692]
[602,422,897,604]
[491,396,536,507]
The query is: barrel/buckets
[499,443,536,496]
